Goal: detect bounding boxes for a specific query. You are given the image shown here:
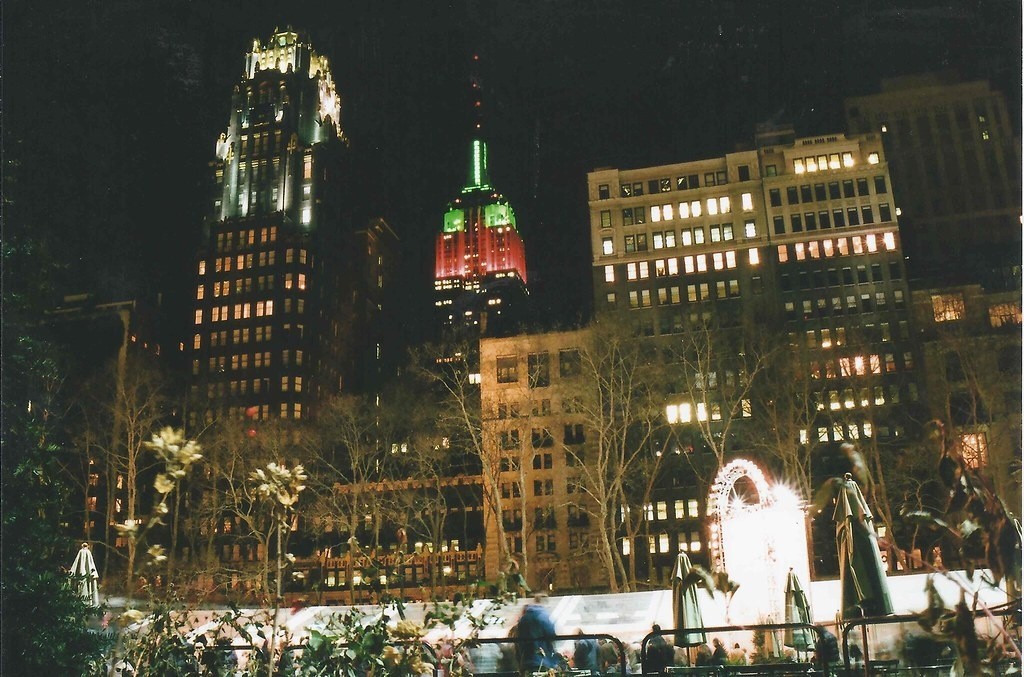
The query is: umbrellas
[765,615,782,663]
[784,567,816,662]
[833,472,894,677]
[69,543,100,609]
[671,549,707,668]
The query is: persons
[554,628,642,677]
[508,593,556,677]
[695,638,729,666]
[730,643,749,666]
[812,626,843,672]
[85,608,503,677]
[646,625,670,672]
